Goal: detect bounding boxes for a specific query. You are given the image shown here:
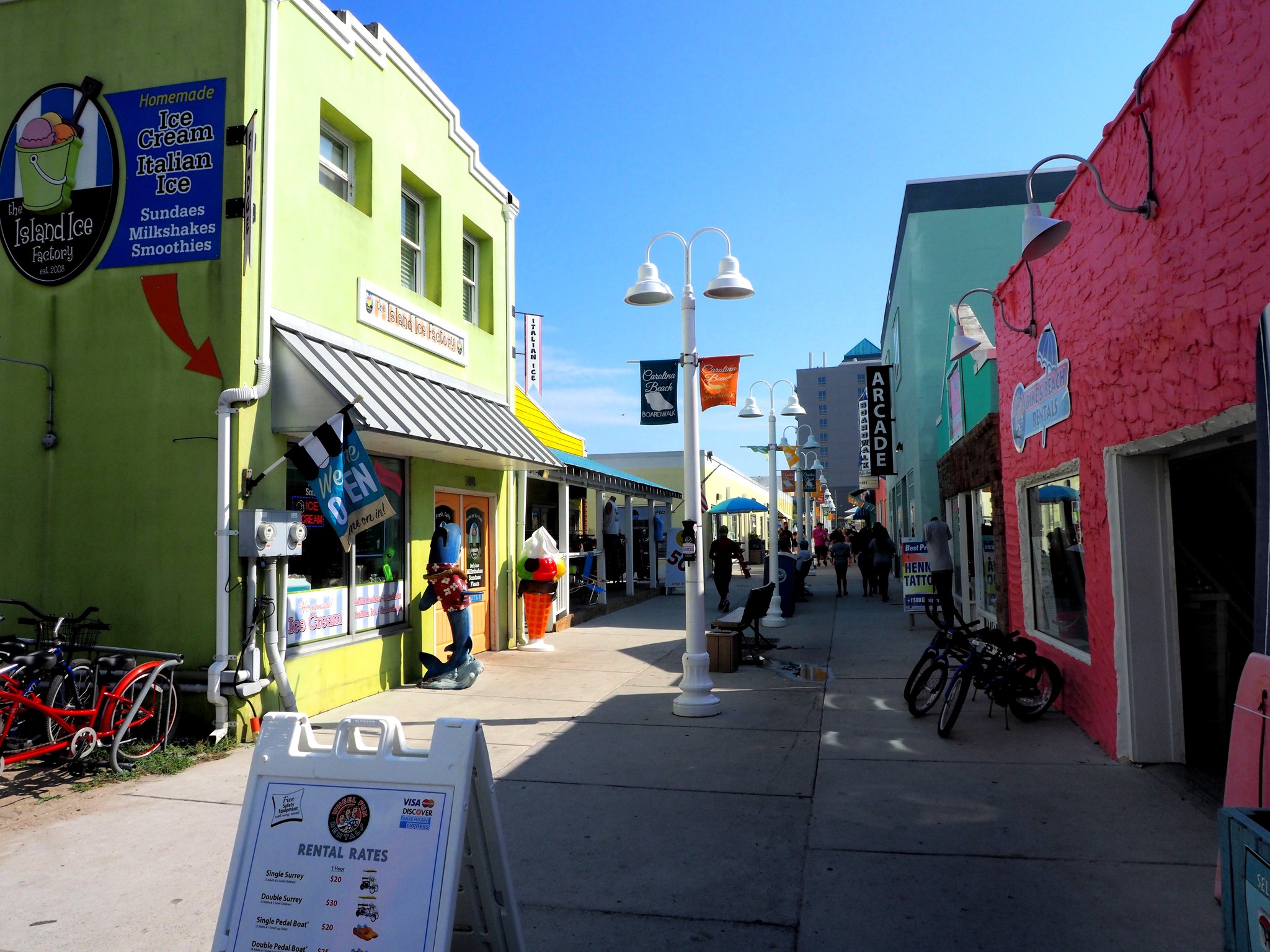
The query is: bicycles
[903,595,1063,738]
[0,593,183,782]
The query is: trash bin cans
[706,630,739,673]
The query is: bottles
[356,565,364,584]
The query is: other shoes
[843,590,848,596]
[836,593,842,598]
[723,600,730,613]
[862,593,867,597]
[718,604,724,609]
[868,592,874,597]
[882,595,889,601]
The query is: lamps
[950,288,1037,362]
[895,441,904,452]
[1020,154,1159,263]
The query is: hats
[817,522,824,526]
[798,540,809,546]
[718,526,728,536]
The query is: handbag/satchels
[873,553,891,569]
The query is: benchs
[710,580,777,661]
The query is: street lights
[739,379,807,628]
[790,451,836,576]
[777,424,820,557]
[625,224,756,722]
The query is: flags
[284,411,397,555]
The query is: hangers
[550,532,594,545]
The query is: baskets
[34,618,100,652]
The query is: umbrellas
[1038,485,1081,531]
[709,497,768,546]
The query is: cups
[472,533,476,547]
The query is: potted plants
[747,531,763,566]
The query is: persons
[824,522,859,566]
[829,533,851,598]
[922,517,956,627]
[709,525,739,613]
[603,496,619,565]
[653,512,664,541]
[777,521,807,554]
[793,540,810,576]
[811,522,828,567]
[852,521,897,603]
